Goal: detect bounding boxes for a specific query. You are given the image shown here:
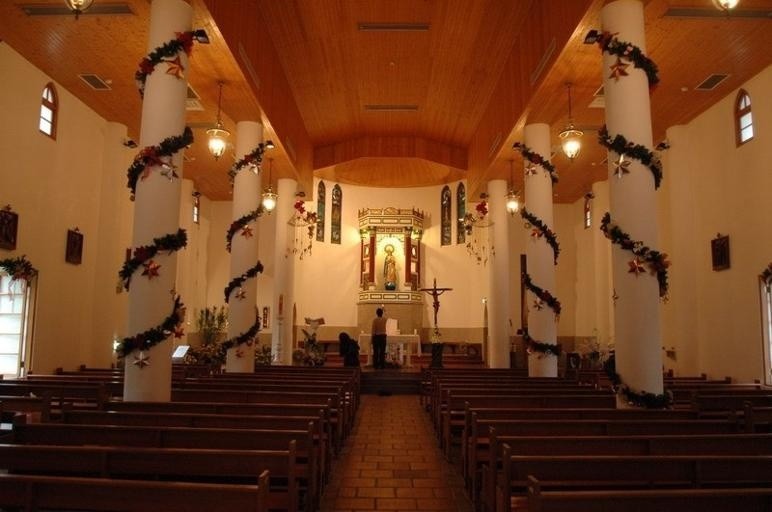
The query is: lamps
[205,79,233,161]
[505,158,521,215]
[261,156,278,216]
[560,79,584,159]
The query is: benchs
[424,365,771,511]
[1,366,359,511]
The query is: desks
[359,332,420,367]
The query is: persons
[338,333,361,368]
[384,249,397,285]
[369,303,390,369]
